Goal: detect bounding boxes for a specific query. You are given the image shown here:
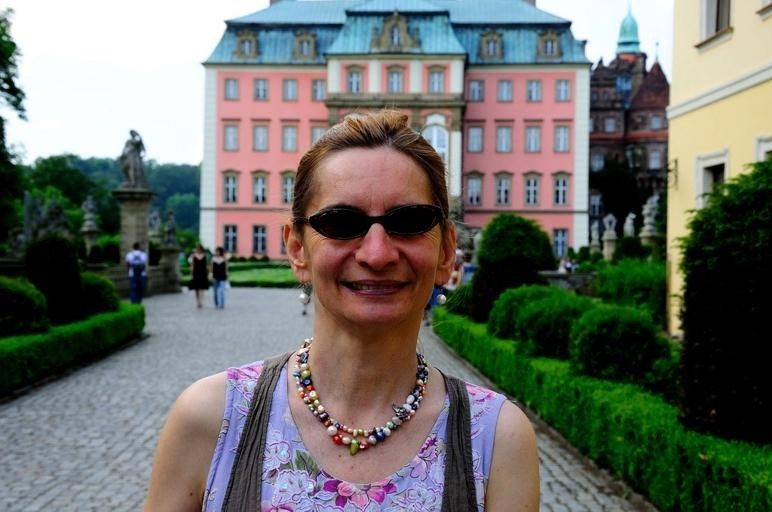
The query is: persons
[188,244,210,307]
[564,257,579,273]
[120,129,145,183]
[125,242,147,304]
[144,108,540,512]
[211,246,230,309]
[425,249,466,326]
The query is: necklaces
[294,336,430,456]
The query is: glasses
[290,201,450,240]
